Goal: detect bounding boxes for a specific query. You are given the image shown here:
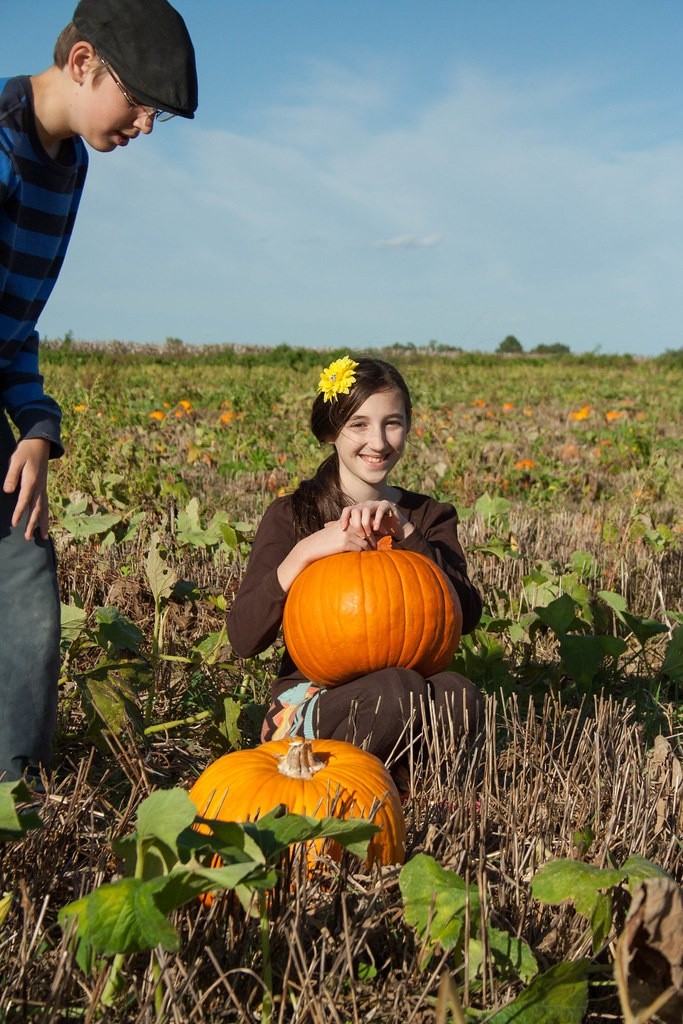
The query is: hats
[73,0,198,120]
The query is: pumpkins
[283,530,463,682]
[71,397,643,473]
[189,739,405,912]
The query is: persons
[228,359,483,796]
[0,0,198,805]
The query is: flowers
[316,355,360,406]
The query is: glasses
[96,53,177,123]
